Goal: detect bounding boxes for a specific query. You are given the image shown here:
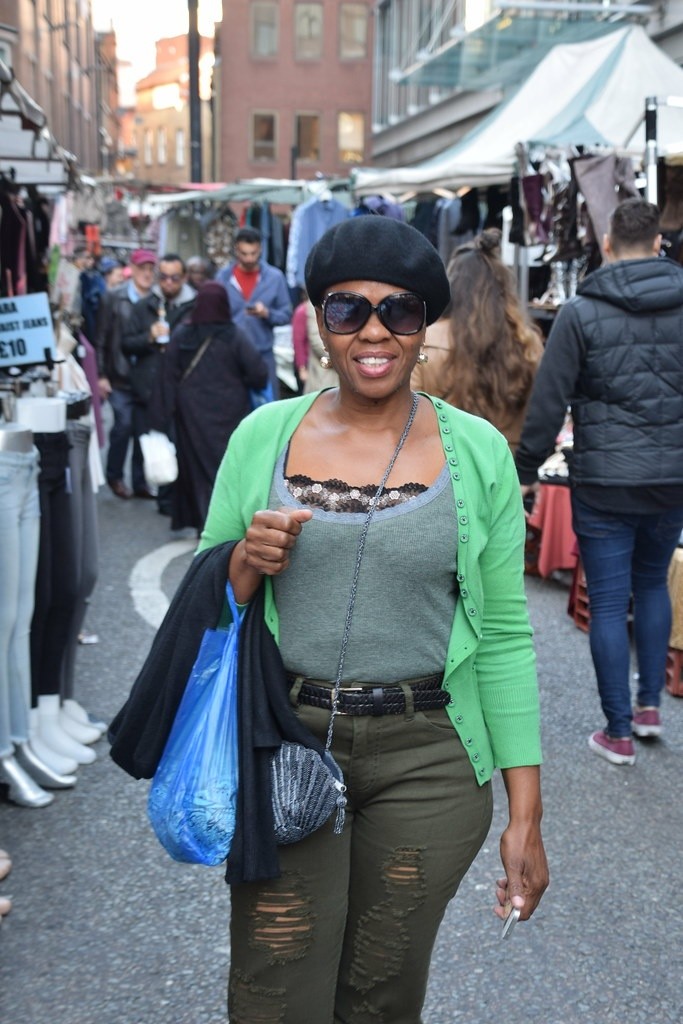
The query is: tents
[116,176,333,278]
[352,20,683,308]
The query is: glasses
[159,272,181,283]
[321,292,426,336]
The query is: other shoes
[108,477,129,498]
[134,488,157,499]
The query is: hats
[305,215,450,326]
[130,248,155,266]
[98,257,117,273]
[123,266,132,279]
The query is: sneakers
[588,731,635,766]
[629,707,660,737]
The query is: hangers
[167,202,192,216]
[397,183,456,205]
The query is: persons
[195,211,551,1024]
[512,198,682,767]
[407,239,551,462]
[287,297,342,395]
[212,226,294,409]
[64,245,269,550]
[0,391,109,917]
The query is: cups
[155,310,170,343]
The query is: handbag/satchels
[139,428,178,485]
[269,741,347,845]
[147,583,247,866]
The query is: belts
[283,675,451,715]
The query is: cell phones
[501,881,521,939]
[246,306,254,310]
